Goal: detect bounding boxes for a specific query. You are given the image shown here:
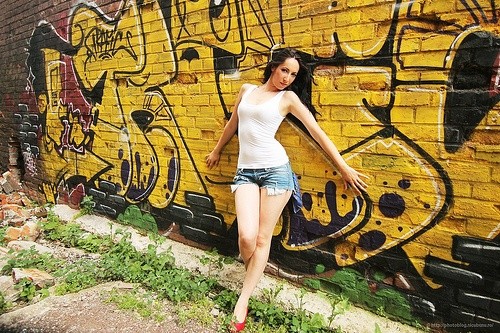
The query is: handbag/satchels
[289,171,303,214]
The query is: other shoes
[229,307,249,333]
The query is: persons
[205,48,370,333]
